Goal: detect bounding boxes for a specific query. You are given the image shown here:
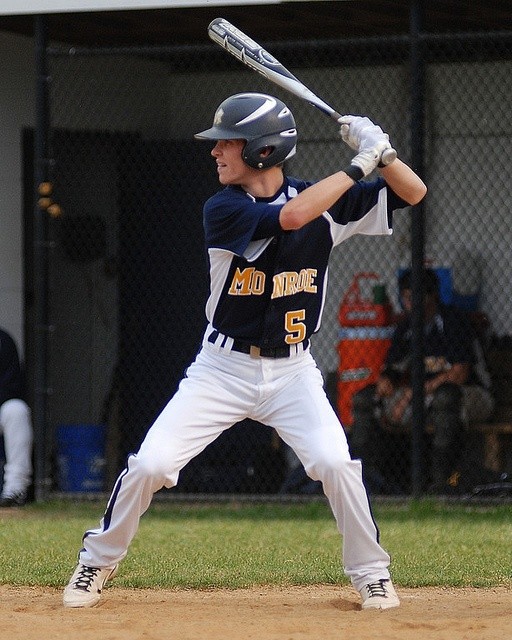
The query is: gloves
[351,126,390,177]
[336,112,389,153]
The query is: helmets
[194,93,298,171]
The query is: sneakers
[359,579,400,610]
[63,563,119,607]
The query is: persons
[351,267,495,493]
[0,327,35,505]
[63,92,428,611]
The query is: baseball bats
[208,17,397,166]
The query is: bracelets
[342,165,365,182]
[377,161,386,168]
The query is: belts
[207,330,309,359]
